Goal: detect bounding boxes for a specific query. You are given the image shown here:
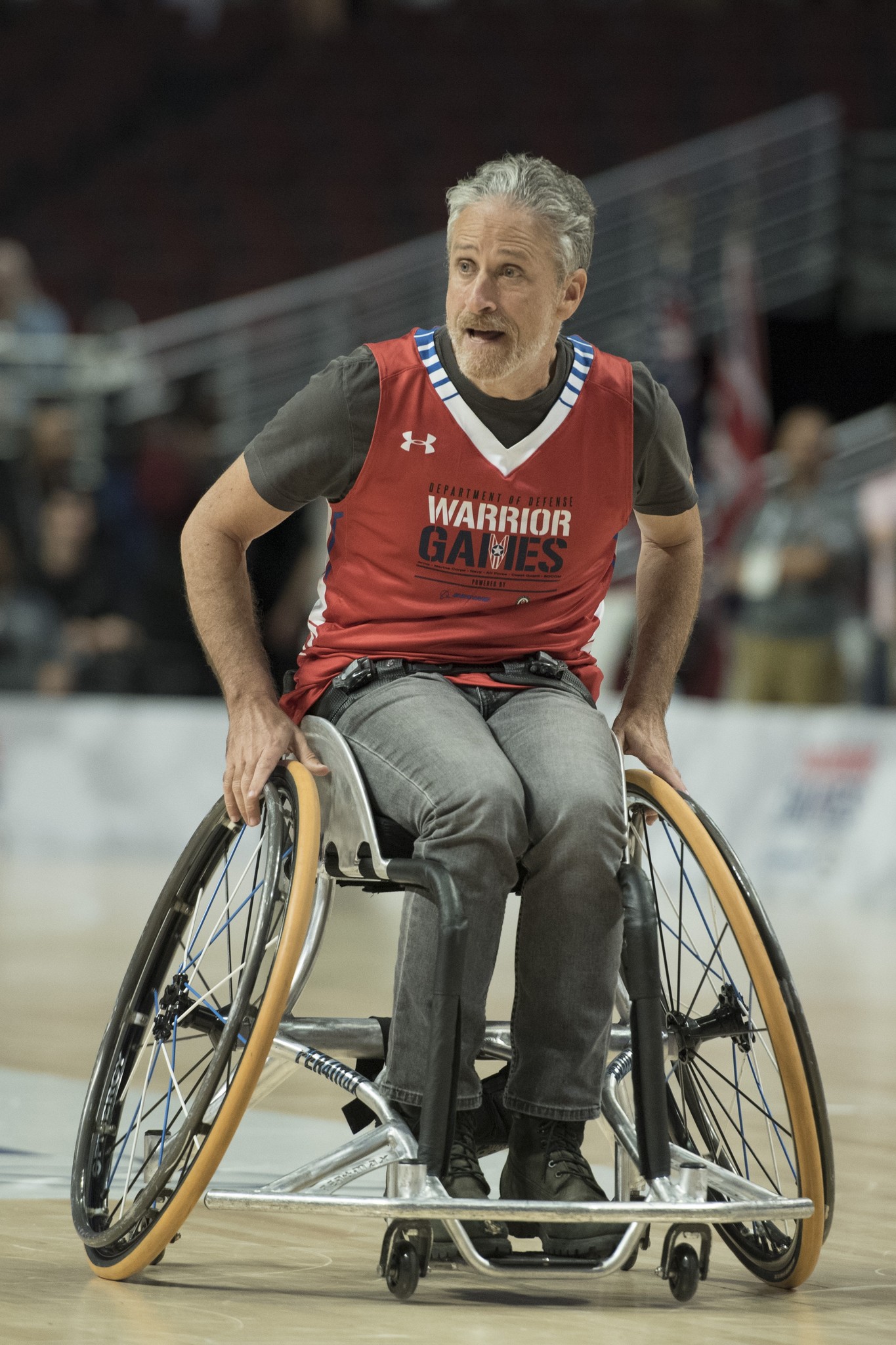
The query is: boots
[413,1119,511,1259]
[499,1117,630,1258]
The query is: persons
[0,239,896,702]
[181,152,703,1261]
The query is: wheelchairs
[70,666,839,1302]
